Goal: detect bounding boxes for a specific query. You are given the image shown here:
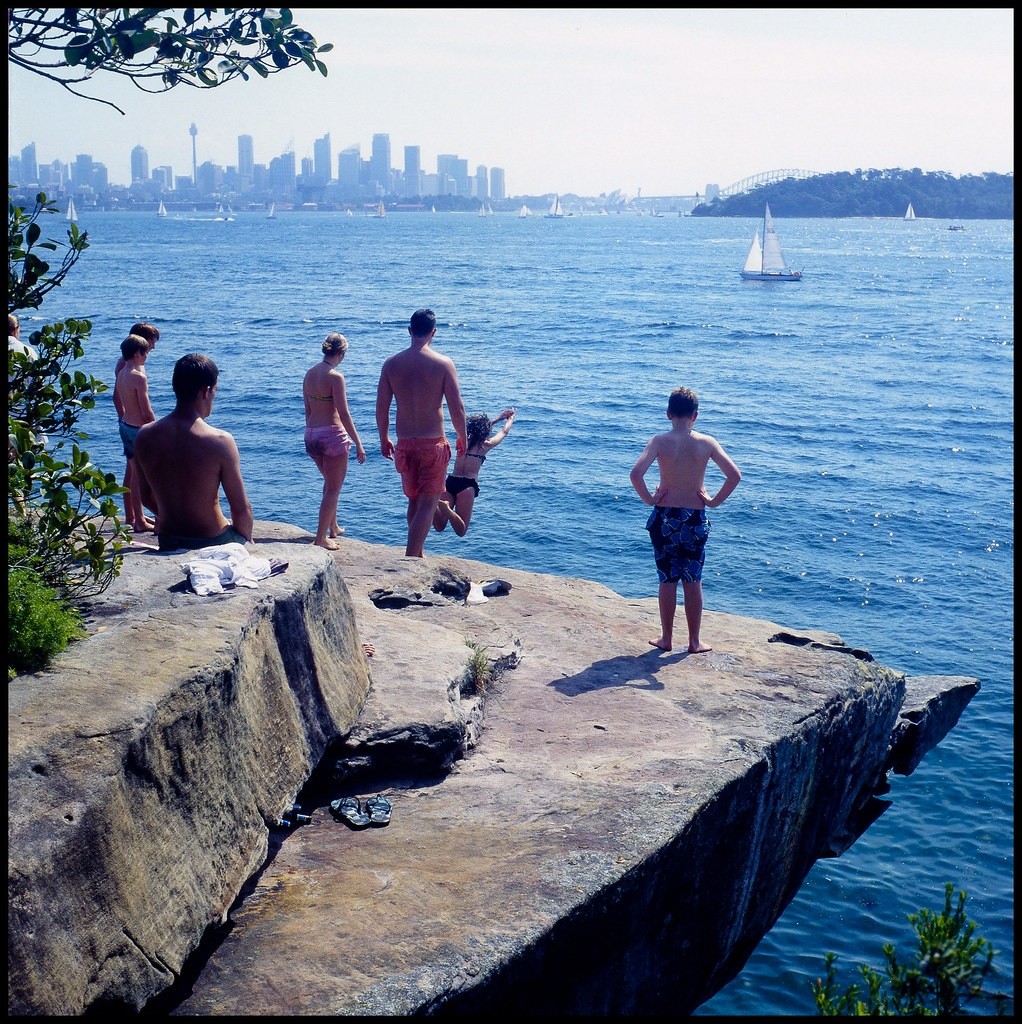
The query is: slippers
[332,794,393,828]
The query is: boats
[947,225,966,230]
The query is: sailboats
[157,200,167,218]
[518,203,531,218]
[476,203,493,218]
[347,210,352,216]
[740,199,803,281]
[266,202,277,219]
[60,197,79,223]
[903,202,917,220]
[542,193,563,218]
[373,200,386,218]
[218,203,237,216]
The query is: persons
[7,313,49,516]
[303,332,366,550]
[117,333,156,532]
[375,308,469,557]
[113,322,159,522]
[136,352,255,550]
[630,386,741,652]
[433,407,514,536]
